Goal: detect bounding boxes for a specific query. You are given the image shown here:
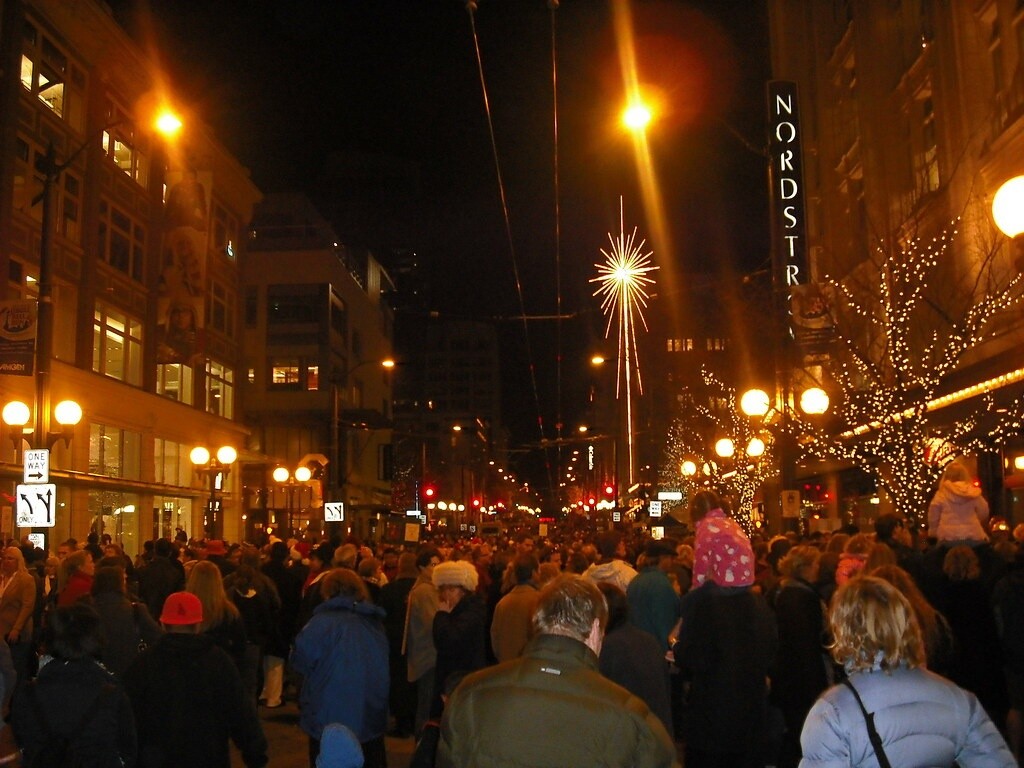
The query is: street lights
[615,88,796,486]
[330,357,397,544]
[272,466,313,538]
[1,104,186,624]
[186,444,237,539]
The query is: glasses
[437,584,463,594]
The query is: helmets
[990,519,1012,532]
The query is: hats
[431,560,478,593]
[295,542,312,559]
[645,542,679,558]
[203,540,228,555]
[311,540,335,565]
[158,592,204,626]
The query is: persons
[0,505,1024,768]
[926,463,990,542]
[91,517,105,535]
[687,492,755,590]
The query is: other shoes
[257,698,267,706]
[267,700,285,708]
[282,692,297,703]
